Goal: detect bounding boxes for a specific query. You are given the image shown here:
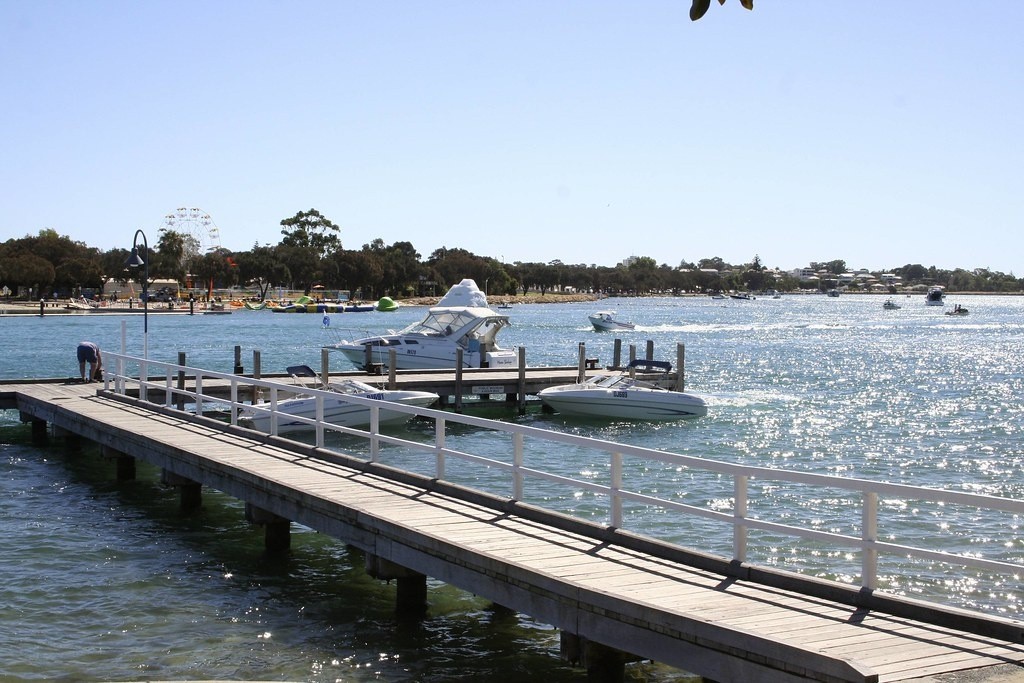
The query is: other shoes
[90,379,99,383]
[82,377,86,382]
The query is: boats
[945,303,970,316]
[498,304,513,309]
[376,296,398,311]
[884,299,901,309]
[712,294,730,301]
[537,376,708,421]
[271,296,375,313]
[827,289,839,297]
[588,311,635,331]
[924,288,944,306]
[319,279,529,372]
[773,290,781,299]
[730,293,756,301]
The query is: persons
[14,287,366,310]
[77,341,102,384]
[599,313,613,322]
[955,304,961,312]
[444,325,453,336]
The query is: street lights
[486,277,490,296]
[124,230,149,400]
